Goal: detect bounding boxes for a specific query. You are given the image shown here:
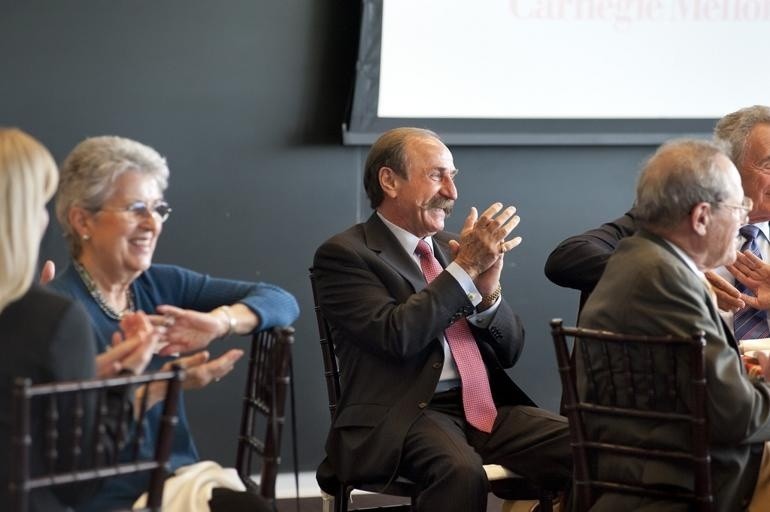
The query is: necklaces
[74,257,141,323]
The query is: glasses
[715,195,754,220]
[93,201,173,223]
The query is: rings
[162,314,173,327]
[213,376,221,383]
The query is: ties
[733,225,768,340]
[416,240,498,434]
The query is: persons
[541,101,769,340]
[1,127,249,510]
[37,130,302,509]
[569,137,770,511]
[305,120,578,510]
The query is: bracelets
[218,304,238,343]
[479,285,504,306]
